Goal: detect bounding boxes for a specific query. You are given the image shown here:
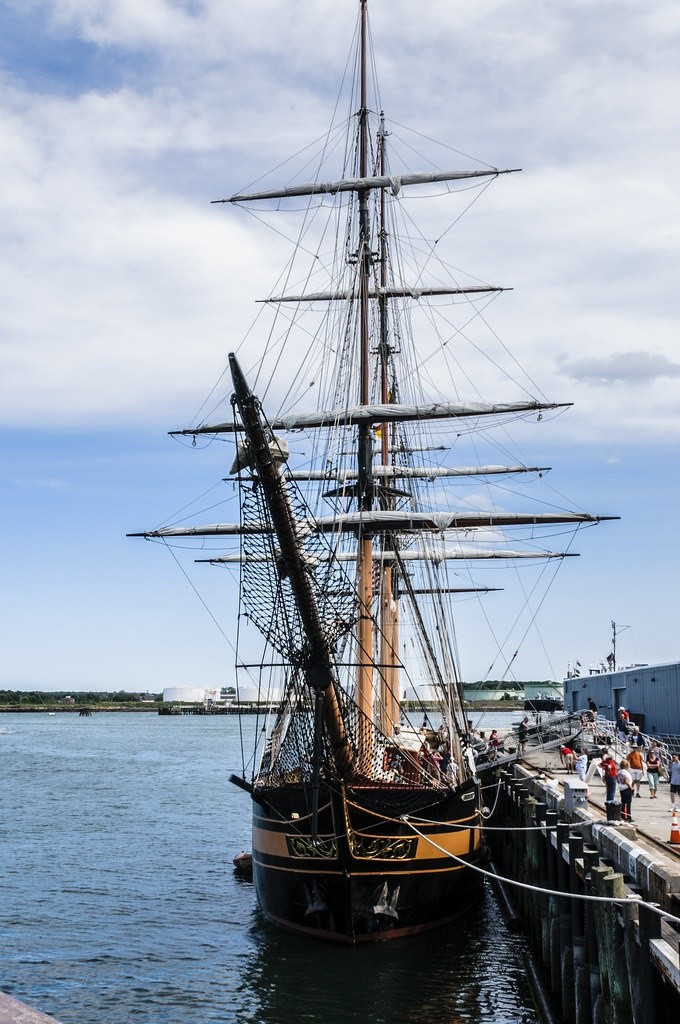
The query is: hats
[618,706,626,711]
[561,745,565,748]
[630,743,639,748]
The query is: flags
[375,391,392,437]
[574,661,582,677]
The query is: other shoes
[636,793,641,798]
[653,796,657,798]
[675,809,680,812]
[650,797,653,799]
[669,808,674,812]
[629,820,634,822]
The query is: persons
[560,744,574,774]
[389,698,680,824]
[607,653,614,671]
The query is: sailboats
[127,1,621,942]
